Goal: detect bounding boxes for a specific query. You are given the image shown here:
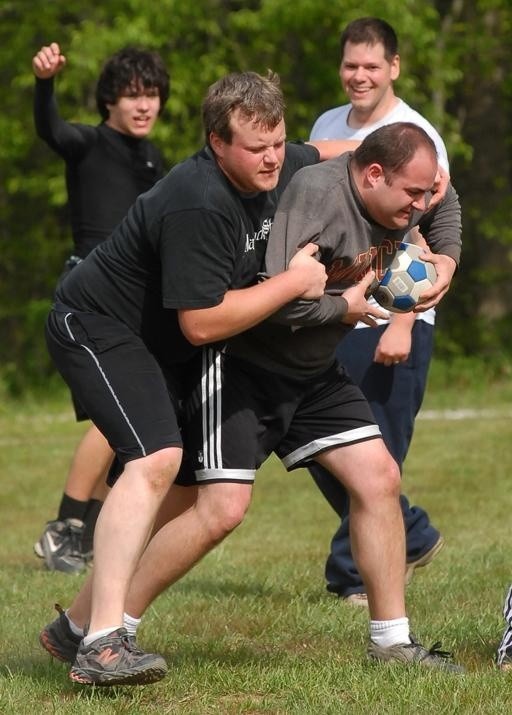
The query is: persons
[39,69,450,686]
[34,42,174,574]
[121,123,465,676]
[309,17,450,607]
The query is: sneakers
[32,515,95,579]
[37,601,169,689]
[365,634,465,680]
[401,534,448,590]
[345,592,372,610]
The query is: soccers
[369,242,436,315]
